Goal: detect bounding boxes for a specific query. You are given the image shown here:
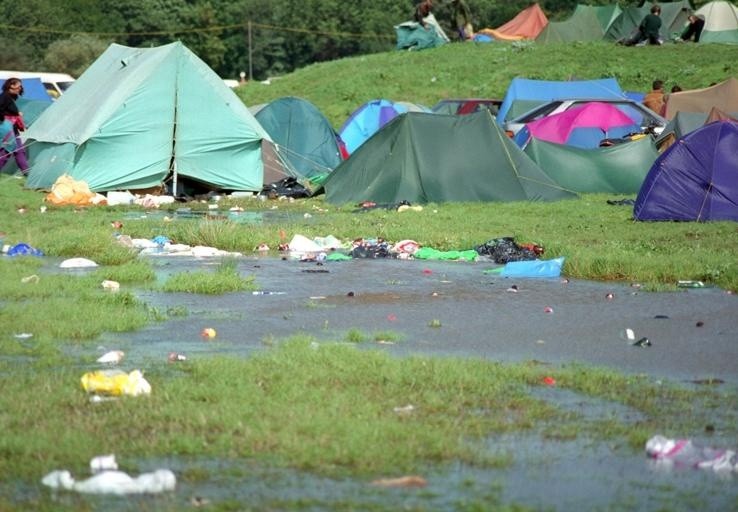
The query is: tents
[391,12,450,51]
[490,0,738,39]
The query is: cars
[434,100,502,117]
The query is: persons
[411,0,433,30]
[448,0,472,42]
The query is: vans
[1,69,75,97]
